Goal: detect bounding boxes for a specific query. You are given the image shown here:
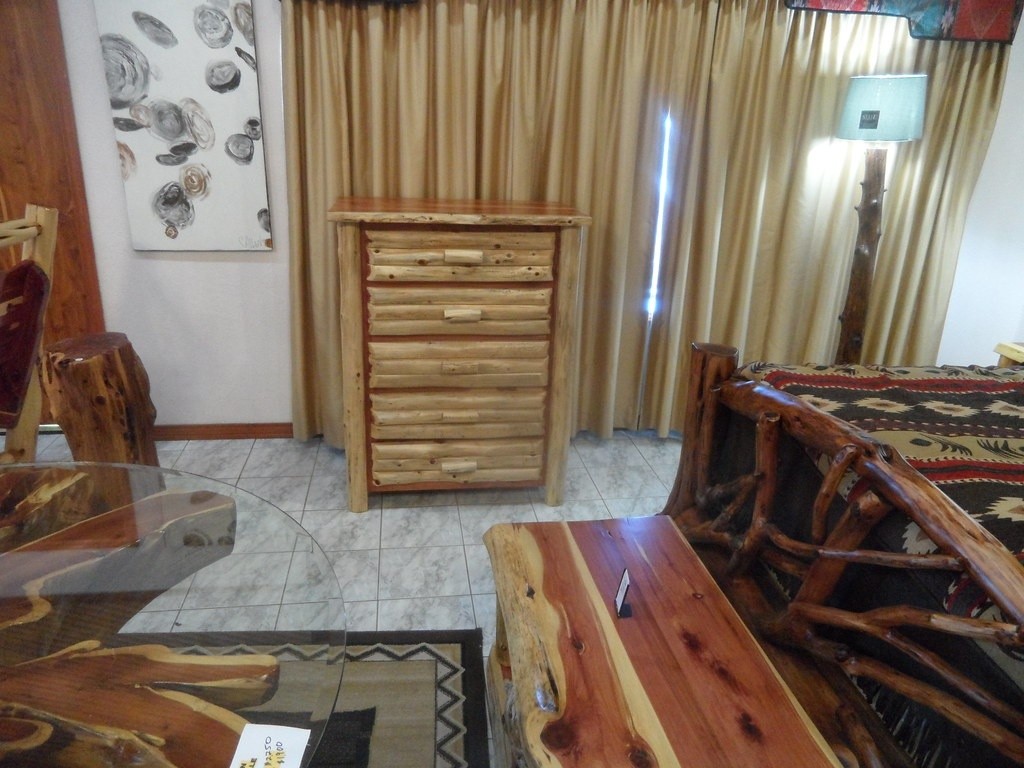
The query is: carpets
[112,627,489,768]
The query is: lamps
[834,74,929,369]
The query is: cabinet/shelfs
[328,196,592,514]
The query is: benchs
[481,343,1024,768]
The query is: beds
[714,359,1024,768]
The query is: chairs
[0,200,61,470]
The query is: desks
[0,461,347,768]
